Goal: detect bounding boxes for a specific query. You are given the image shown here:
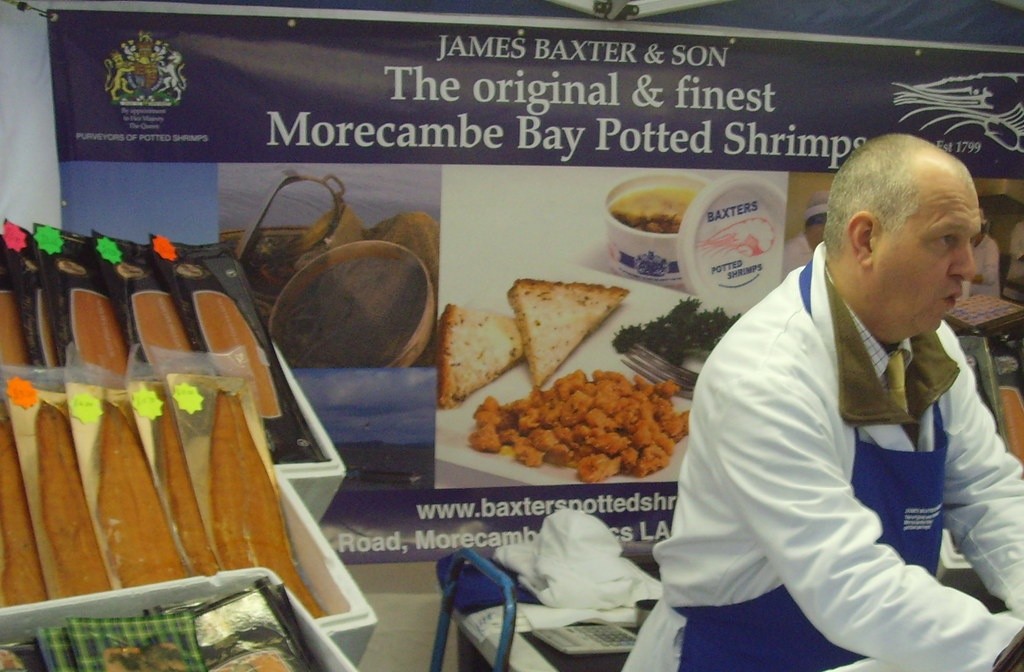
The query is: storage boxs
[0,342,379,672]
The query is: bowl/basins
[604,171,712,287]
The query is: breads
[434,304,524,408]
[508,278,630,390]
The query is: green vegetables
[611,296,745,371]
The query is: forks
[620,343,699,392]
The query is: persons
[625,133,1024,672]
[1004,221,1024,305]
[969,208,1003,299]
[779,189,831,286]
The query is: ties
[886,348,908,412]
